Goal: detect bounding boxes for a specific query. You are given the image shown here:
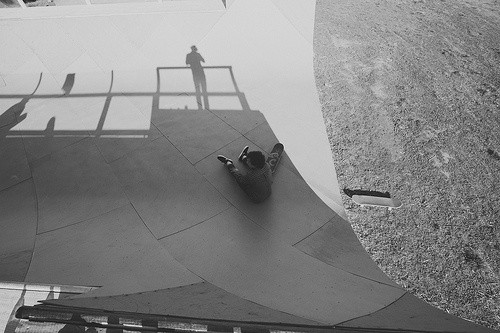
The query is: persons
[216,145,272,204]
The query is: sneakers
[238,145,249,160]
[217,154,233,165]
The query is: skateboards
[351,193,403,211]
